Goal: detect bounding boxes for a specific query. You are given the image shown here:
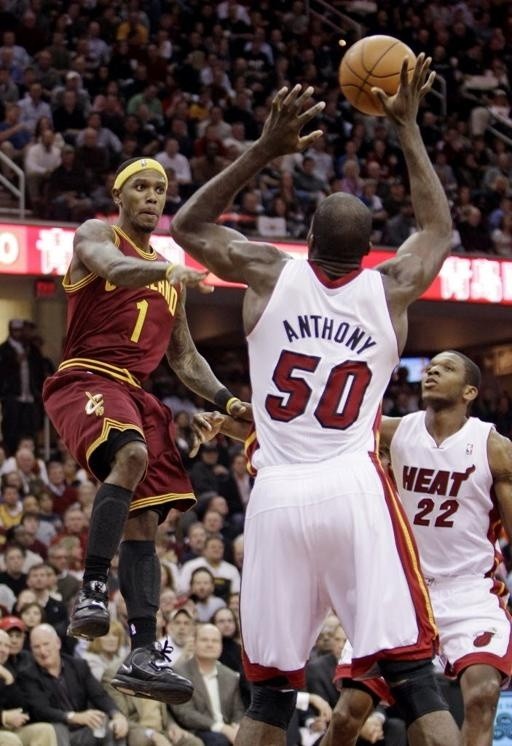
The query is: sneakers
[66,579,110,642]
[111,648,194,705]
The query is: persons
[0,319,512,746]
[44,157,253,704]
[170,53,466,746]
[2,0,512,259]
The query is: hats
[0,617,27,631]
[169,607,193,622]
[66,70,81,81]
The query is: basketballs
[340,35,416,117]
